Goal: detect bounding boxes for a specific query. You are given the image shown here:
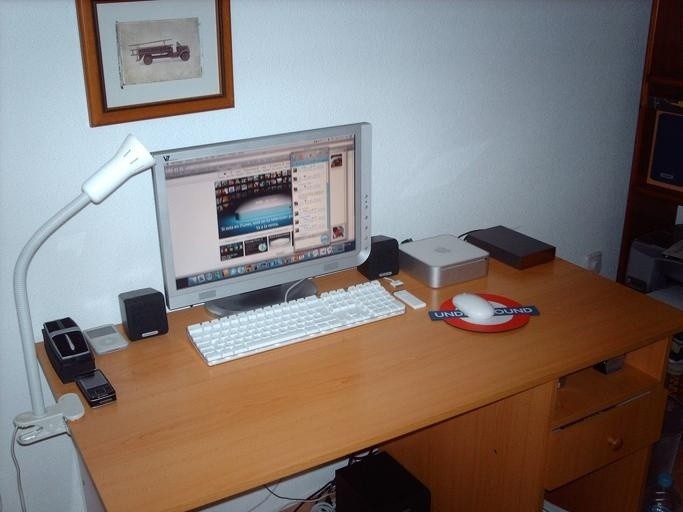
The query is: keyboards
[185,278,405,366]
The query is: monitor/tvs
[150,122,372,317]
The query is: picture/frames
[76,0,237,129]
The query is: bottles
[643,471,683,512]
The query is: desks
[34,256,683,512]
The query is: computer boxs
[335,451,431,512]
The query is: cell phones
[73,367,117,409]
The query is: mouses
[451,291,495,321]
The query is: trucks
[128,39,190,65]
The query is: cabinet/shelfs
[615,0,683,282]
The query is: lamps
[10,132,158,447]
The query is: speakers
[118,287,168,341]
[356,235,399,281]
